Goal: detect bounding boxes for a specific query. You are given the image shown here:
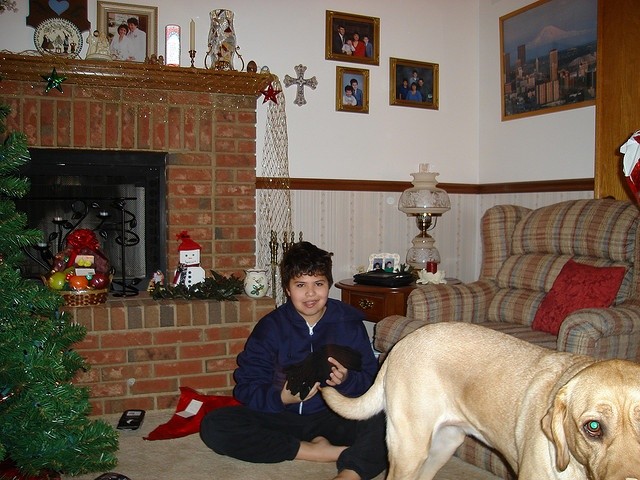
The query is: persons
[333,25,372,58]
[385,261,391,272]
[343,78,363,106]
[374,262,380,270]
[400,71,426,103]
[111,16,146,63]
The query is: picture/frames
[498,0,595,122]
[324,9,380,66]
[335,65,370,114]
[389,56,440,111]
[96,0,158,61]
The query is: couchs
[373,198,640,373]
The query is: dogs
[321,316,640,480]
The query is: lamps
[366,252,401,273]
[397,164,452,271]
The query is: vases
[243,269,269,298]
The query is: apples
[48,272,67,291]
[89,275,110,290]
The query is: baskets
[40,269,115,308]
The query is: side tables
[334,278,463,324]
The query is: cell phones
[117,409,146,430]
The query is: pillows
[530,258,628,336]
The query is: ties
[354,90,355,96]
[343,36,344,43]
